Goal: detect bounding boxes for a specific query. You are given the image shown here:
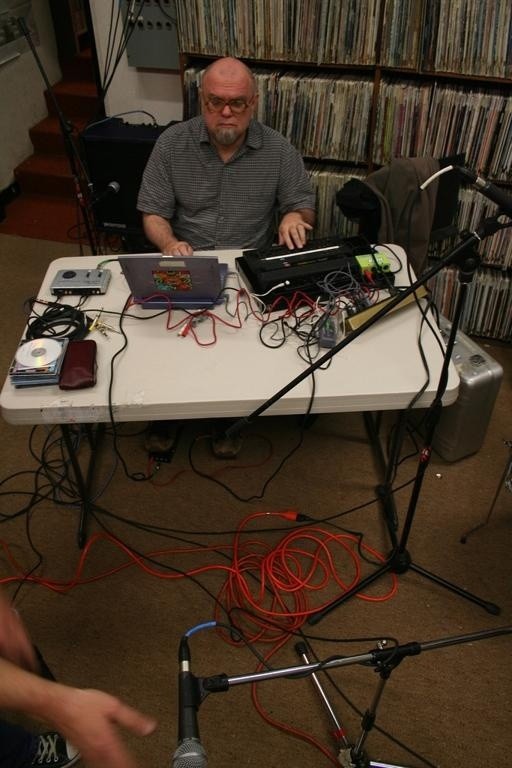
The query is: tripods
[202,627,512,768]
[225,208,512,627]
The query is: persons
[0,590,157,768]
[136,56,317,460]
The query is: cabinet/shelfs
[176,0,512,344]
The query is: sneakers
[212,418,243,457]
[143,421,178,452]
[31,732,80,767]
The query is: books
[423,258,512,341]
[183,69,203,119]
[175,0,512,80]
[302,161,512,269]
[250,68,512,182]
[9,337,69,385]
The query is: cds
[15,338,62,367]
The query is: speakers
[80,124,167,236]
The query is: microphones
[86,181,120,211]
[452,165,512,219]
[169,636,208,768]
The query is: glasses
[202,90,254,113]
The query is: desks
[1,244,461,548]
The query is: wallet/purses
[59,340,98,389]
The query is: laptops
[117,255,228,309]
